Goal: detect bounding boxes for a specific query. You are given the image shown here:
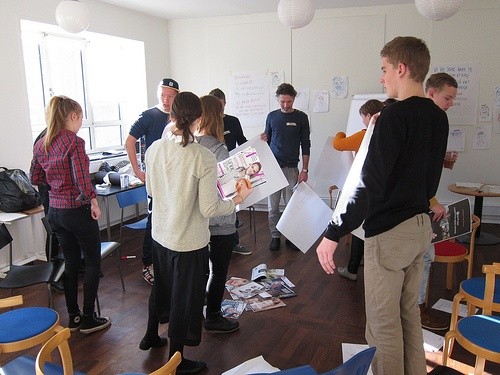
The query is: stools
[328,185,351,245]
[425,212,500,375]
[0,295,181,375]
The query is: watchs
[303,169,308,172]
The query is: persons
[332,99,384,278]
[261,83,311,252]
[420,73,458,330]
[138,91,253,375]
[124,78,179,286]
[315,36,449,375]
[218,162,261,189]
[192,96,240,334]
[210,88,252,256]
[29,95,111,335]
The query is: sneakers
[233,243,251,255]
[80,314,111,335]
[68,313,83,332]
[142,264,154,285]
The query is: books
[216,146,266,201]
[222,263,296,319]
[429,198,472,244]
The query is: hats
[160,79,179,92]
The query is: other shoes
[168,359,206,375]
[270,238,280,249]
[419,303,448,330]
[139,336,167,350]
[338,266,357,280]
[286,239,298,250]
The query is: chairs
[0,187,148,315]
[251,347,377,375]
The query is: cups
[444,151,458,169]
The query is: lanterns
[278,0,315,30]
[415,0,463,20]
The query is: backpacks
[0,166,42,212]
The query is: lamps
[55,0,89,32]
[279,1,316,28]
[415,0,461,20]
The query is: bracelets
[238,195,244,203]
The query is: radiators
[95,191,136,231]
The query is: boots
[205,308,239,333]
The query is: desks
[94,183,145,257]
[446,183,500,247]
[0,206,44,225]
